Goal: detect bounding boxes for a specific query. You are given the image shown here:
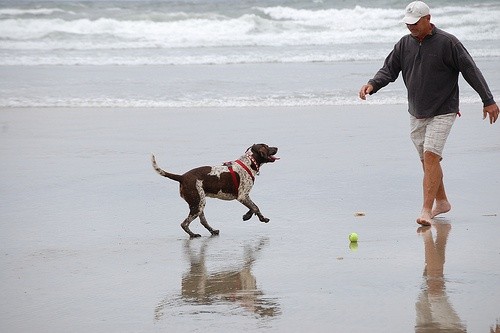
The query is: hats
[402,1,431,24]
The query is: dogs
[151,144,280,238]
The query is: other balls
[348,233,359,242]
[349,242,358,249]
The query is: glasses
[406,18,422,25]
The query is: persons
[360,1,499,226]
